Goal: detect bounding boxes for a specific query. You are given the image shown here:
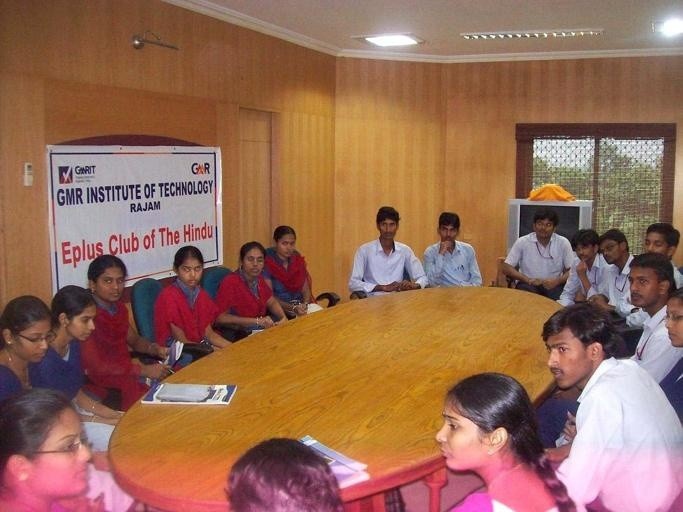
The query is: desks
[107,286,565,512]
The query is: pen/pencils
[158,361,175,374]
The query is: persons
[80,254,179,410]
[436,372,575,511]
[215,242,286,341]
[40,286,126,426]
[589,229,636,315]
[1,295,145,512]
[541,304,683,512]
[265,226,318,315]
[0,389,95,511]
[627,253,683,383]
[347,206,427,297]
[657,289,683,422]
[502,209,574,299]
[556,228,606,306]
[423,212,482,287]
[154,245,232,365]
[227,439,341,512]
[643,224,682,291]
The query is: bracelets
[256,314,260,329]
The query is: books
[299,435,371,489]
[161,340,184,365]
[142,383,238,406]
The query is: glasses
[13,329,58,344]
[21,438,101,458]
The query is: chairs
[130,278,252,369]
[204,267,230,301]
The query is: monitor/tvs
[506,199,594,257]
